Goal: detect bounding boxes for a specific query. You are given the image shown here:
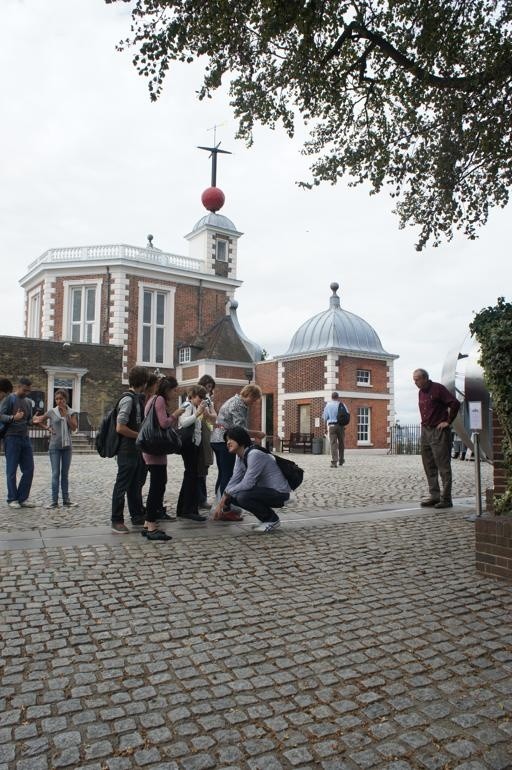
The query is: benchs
[277,430,315,457]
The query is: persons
[322,392,349,468]
[0,377,43,510]
[212,426,292,533]
[413,369,462,510]
[138,373,268,523]
[36,388,78,509]
[110,366,159,534]
[140,376,186,541]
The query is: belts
[329,422,338,425]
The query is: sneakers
[421,497,453,509]
[109,500,281,542]
[7,499,36,509]
[42,499,78,509]
[330,459,345,467]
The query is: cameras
[204,398,210,407]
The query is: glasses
[197,394,204,399]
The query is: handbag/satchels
[134,394,183,454]
[174,407,196,454]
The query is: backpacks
[337,402,350,426]
[243,444,305,491]
[0,393,17,439]
[95,392,136,459]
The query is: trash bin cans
[312,437,322,455]
